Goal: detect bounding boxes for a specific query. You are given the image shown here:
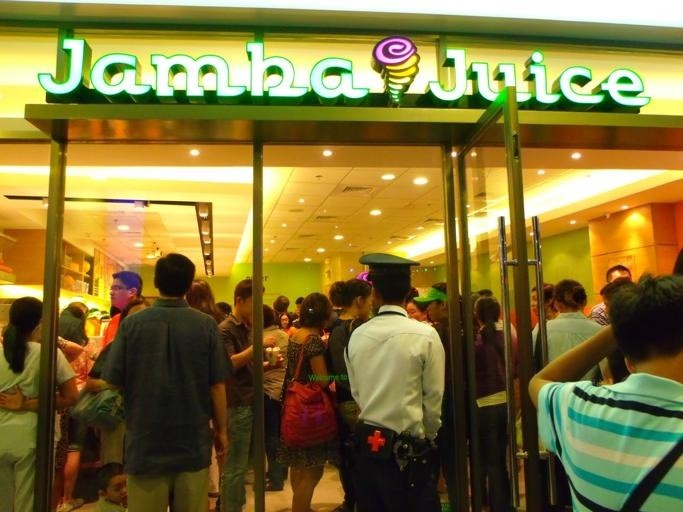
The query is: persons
[0,245,683,512]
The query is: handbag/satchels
[67,387,127,429]
[356,422,396,459]
[338,401,361,433]
[281,379,340,448]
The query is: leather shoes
[253,481,282,491]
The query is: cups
[266,346,280,365]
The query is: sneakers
[63,498,84,511]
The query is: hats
[359,252,420,280]
[413,287,448,303]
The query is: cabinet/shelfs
[3,229,94,294]
